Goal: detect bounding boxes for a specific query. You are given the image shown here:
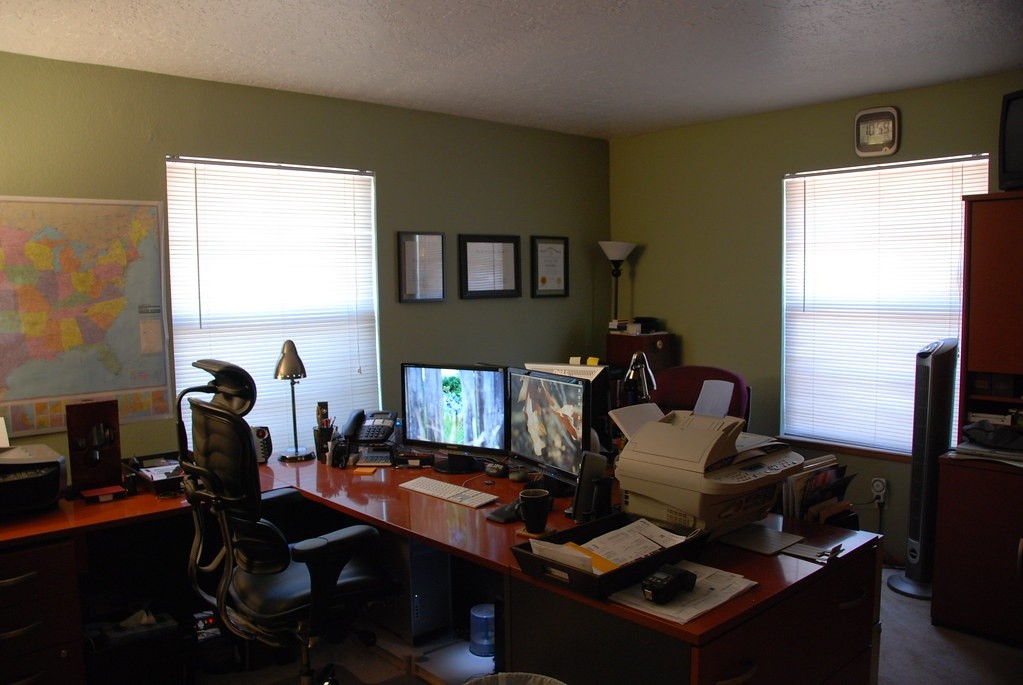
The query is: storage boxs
[510,512,710,600]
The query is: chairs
[174,359,386,685]
[649,365,752,434]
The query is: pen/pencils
[321,416,336,428]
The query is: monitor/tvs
[997,89,1023,191]
[401,361,613,498]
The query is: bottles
[624,364,651,407]
[608,379,626,451]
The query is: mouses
[505,501,519,512]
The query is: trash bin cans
[463,672,567,685]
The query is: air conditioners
[887,337,959,601]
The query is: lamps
[623,350,657,403]
[597,240,638,331]
[274,340,315,463]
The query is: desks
[0,451,884,685]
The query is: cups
[514,489,551,534]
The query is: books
[780,454,855,525]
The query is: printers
[609,379,806,541]
[0,443,68,523]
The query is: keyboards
[399,477,499,508]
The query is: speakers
[568,450,608,525]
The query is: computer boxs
[362,529,455,647]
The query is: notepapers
[352,467,376,474]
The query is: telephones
[342,409,398,444]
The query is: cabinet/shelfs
[931,192,1022,653]
[606,331,677,409]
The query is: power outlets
[871,478,886,504]
